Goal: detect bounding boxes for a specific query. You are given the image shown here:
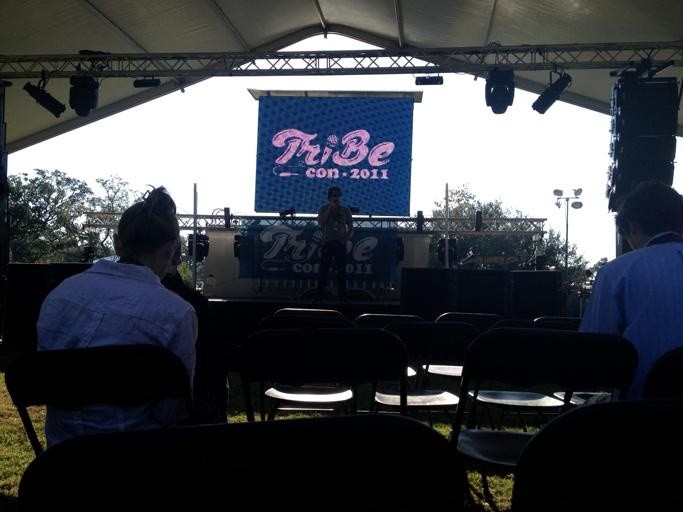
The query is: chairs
[510,398,681,512]
[202,298,680,477]
[18,412,473,512]
[7,343,212,457]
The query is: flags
[248,88,425,218]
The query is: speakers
[457,268,512,326]
[400,267,458,320]
[512,270,562,327]
[608,79,679,214]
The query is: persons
[31,184,198,451]
[314,185,355,301]
[162,237,224,424]
[578,176,682,408]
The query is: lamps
[21,45,573,118]
